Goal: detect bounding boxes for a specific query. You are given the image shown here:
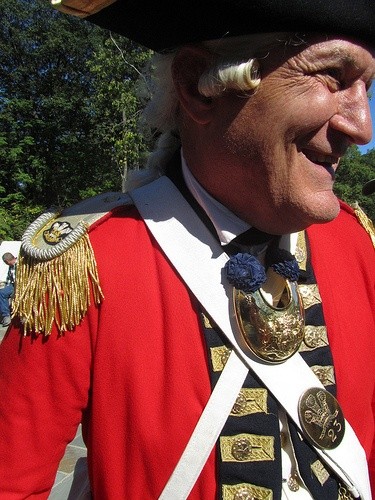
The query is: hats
[51,0,375,55]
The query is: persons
[0,0,375,500]
[0,253,18,328]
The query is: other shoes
[1,316,11,327]
